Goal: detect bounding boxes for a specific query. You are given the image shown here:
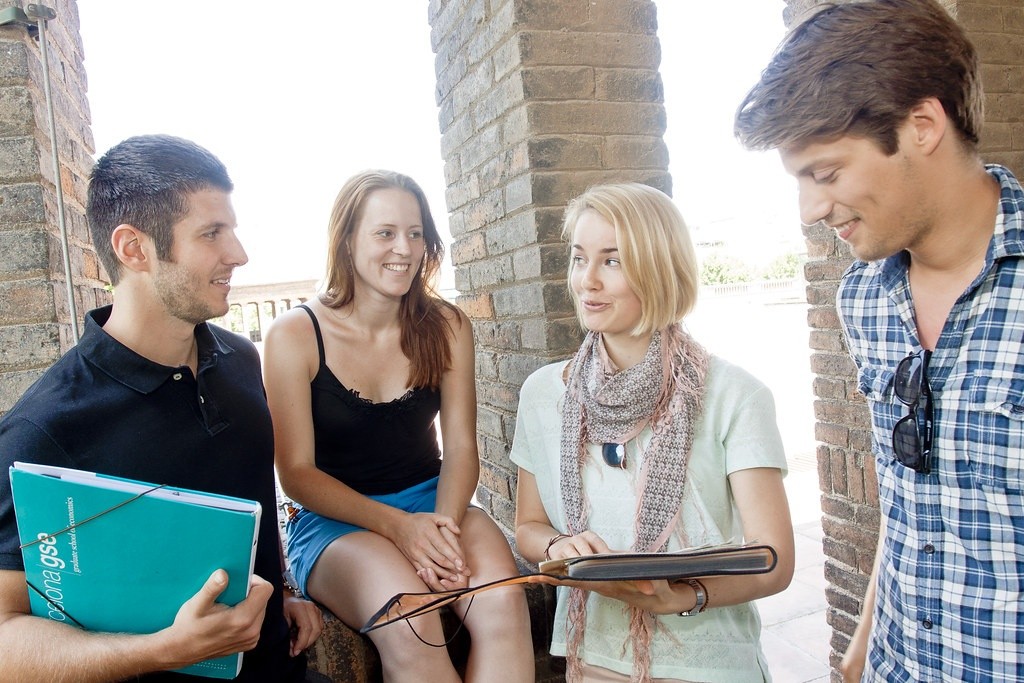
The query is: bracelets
[696,579,709,612]
[544,533,573,560]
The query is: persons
[0,133,333,683]
[735,0,1024,683]
[264,171,535,683]
[509,182,796,683]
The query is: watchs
[676,578,703,617]
[830,669,846,683]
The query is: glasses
[893,348,936,471]
[602,443,627,472]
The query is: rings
[585,590,591,593]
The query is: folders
[8,461,264,679]
